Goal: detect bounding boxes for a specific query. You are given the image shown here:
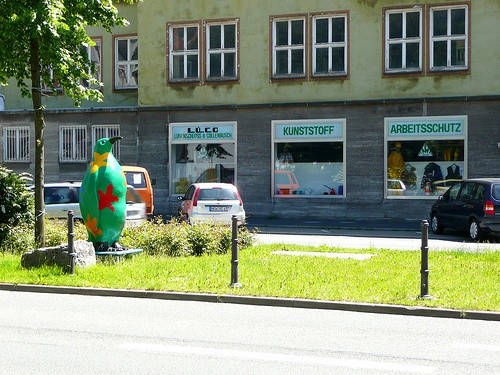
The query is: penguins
[78,137,128,253]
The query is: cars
[177,182,246,230]
[429,176,500,242]
[386,176,462,197]
[194,168,343,197]
[23,181,146,230]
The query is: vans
[119,164,154,221]
